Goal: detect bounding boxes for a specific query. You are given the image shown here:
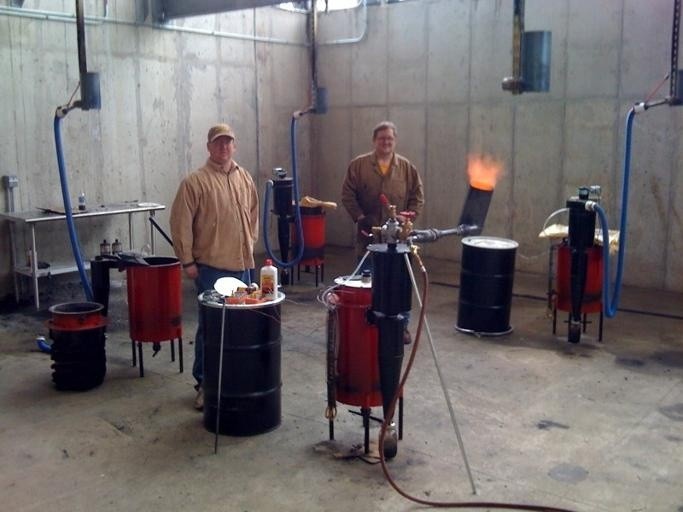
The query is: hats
[208,123,235,143]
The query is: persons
[341,121,425,344]
[170,123,259,411]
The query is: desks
[1,201,166,310]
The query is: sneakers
[194,392,205,410]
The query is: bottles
[259,259,278,300]
[78,190,85,210]
[99,239,110,256]
[112,239,122,255]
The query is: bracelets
[182,261,195,268]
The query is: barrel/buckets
[198,287,285,436]
[49,304,108,391]
[455,236,519,334]
